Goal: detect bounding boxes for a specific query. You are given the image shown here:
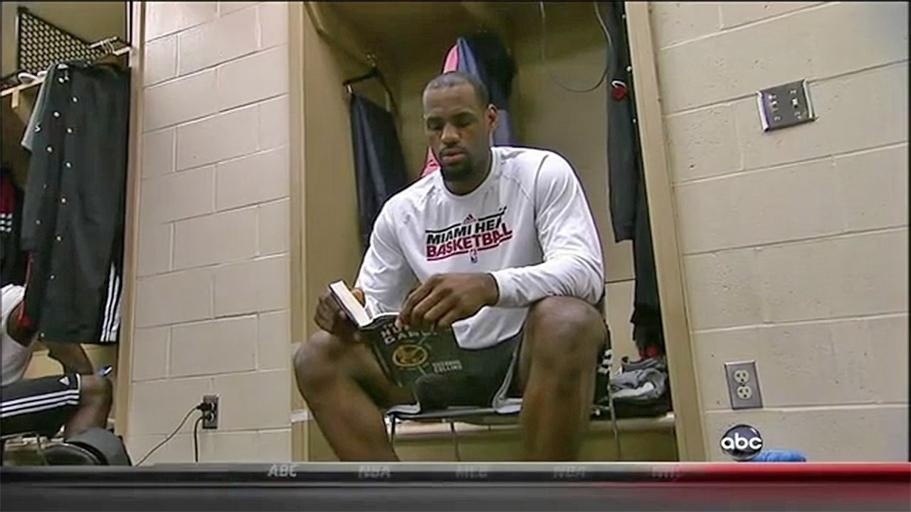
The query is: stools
[0,431,53,465]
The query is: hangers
[344,53,401,121]
[100,38,116,56]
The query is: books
[326,277,466,389]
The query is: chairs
[389,384,623,461]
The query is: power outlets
[202,395,218,427]
[724,362,763,409]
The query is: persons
[2,250,114,445]
[292,67,612,461]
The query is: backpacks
[2,427,131,466]
